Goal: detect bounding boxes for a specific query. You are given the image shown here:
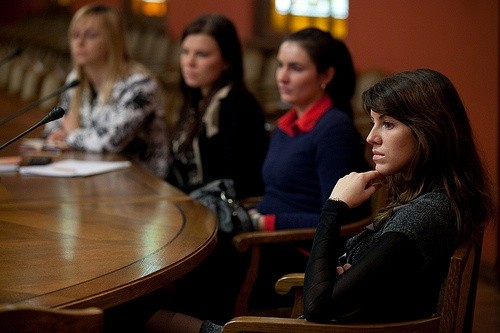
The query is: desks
[0,88,220,312]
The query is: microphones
[0,80,80,126]
[0,107,65,150]
[0,48,24,65]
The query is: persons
[165,13,271,196]
[140,68,494,333]
[36,3,169,178]
[170,27,373,333]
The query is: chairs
[218,100,495,333]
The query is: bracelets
[253,212,261,229]
[329,197,352,210]
